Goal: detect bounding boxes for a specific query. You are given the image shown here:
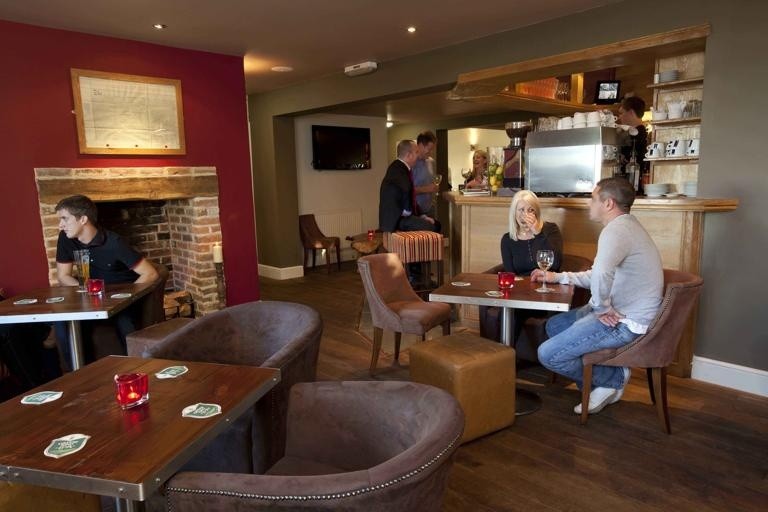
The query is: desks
[345,232,383,274]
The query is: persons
[409,130,442,275]
[530,176,665,416]
[38,194,162,375]
[379,138,436,286]
[486,190,563,366]
[1,290,27,391]
[615,95,649,196]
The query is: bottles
[625,147,640,192]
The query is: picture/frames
[70,67,186,155]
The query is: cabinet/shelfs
[643,76,704,162]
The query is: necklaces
[518,228,532,236]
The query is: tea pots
[667,101,686,119]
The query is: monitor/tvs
[312,125,371,170]
[594,80,621,102]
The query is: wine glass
[433,175,443,197]
[461,167,471,189]
[534,249,556,293]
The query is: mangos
[489,163,503,191]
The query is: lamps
[344,61,378,77]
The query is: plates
[460,189,488,196]
[659,69,680,83]
[643,181,698,198]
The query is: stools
[391,230,443,295]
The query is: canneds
[368,229,375,241]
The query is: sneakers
[574,368,631,415]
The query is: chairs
[299,214,340,275]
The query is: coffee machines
[524,128,634,195]
[502,119,534,187]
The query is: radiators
[302,208,362,268]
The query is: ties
[409,168,416,215]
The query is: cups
[653,73,659,83]
[538,109,618,132]
[497,271,515,290]
[113,371,152,411]
[654,113,667,120]
[72,249,92,292]
[86,279,104,296]
[644,138,699,158]
